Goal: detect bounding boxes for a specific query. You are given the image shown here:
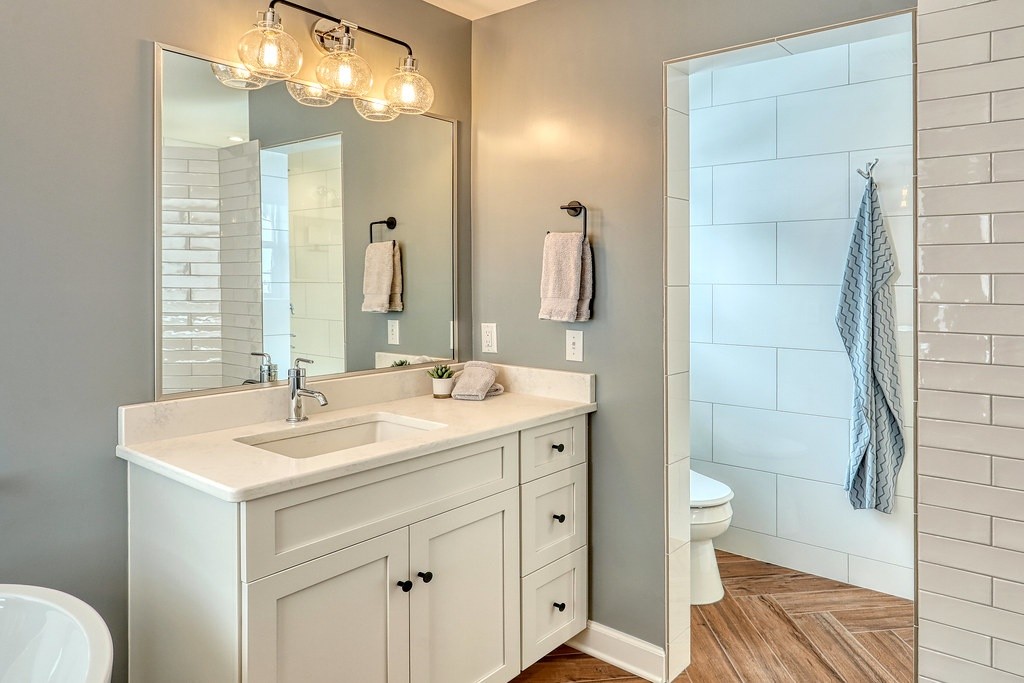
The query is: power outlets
[387,320,399,345]
[566,330,584,362]
[481,322,497,354]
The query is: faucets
[242,352,277,385]
[285,357,328,423]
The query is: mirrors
[151,42,459,400]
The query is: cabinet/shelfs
[128,414,589,683]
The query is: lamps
[353,99,401,121]
[238,0,435,116]
[212,62,271,89]
[285,81,341,108]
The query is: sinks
[0,584,114,683]
[231,412,449,460]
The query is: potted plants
[390,360,411,368]
[427,364,455,399]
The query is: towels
[410,355,442,366]
[450,361,505,401]
[361,241,404,314]
[834,178,906,516]
[538,232,593,324]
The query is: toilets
[690,469,734,605]
